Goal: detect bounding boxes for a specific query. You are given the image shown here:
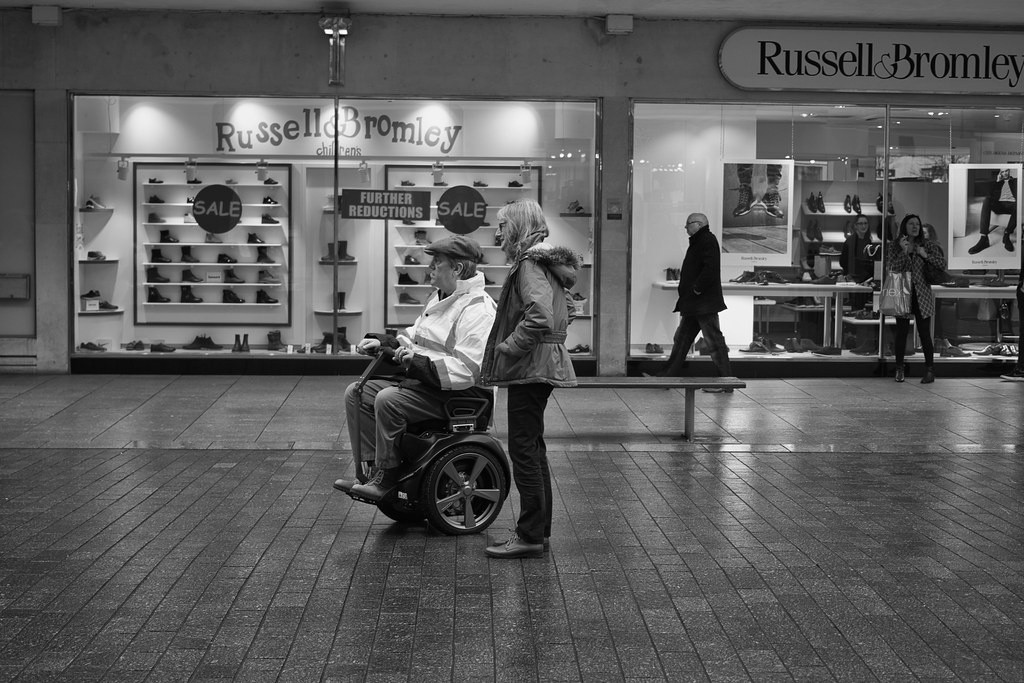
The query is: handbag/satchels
[879,270,911,316]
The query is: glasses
[499,221,509,232]
[686,221,701,225]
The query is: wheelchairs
[347,346,512,536]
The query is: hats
[424,234,482,263]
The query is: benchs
[576,377,746,441]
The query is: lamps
[319,17,353,85]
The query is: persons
[968,168,1018,255]
[481,200,580,560]
[1000,269,1024,381]
[642,213,733,393]
[886,213,945,384]
[839,215,874,311]
[333,236,498,502]
[731,164,784,219]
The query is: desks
[653,282,873,348]
[914,285,1017,349]
[779,304,851,345]
[834,316,914,356]
[755,300,777,334]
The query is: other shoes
[566,200,591,353]
[985,278,1008,286]
[940,276,969,288]
[396,175,523,304]
[880,194,895,214]
[729,191,879,356]
[1002,232,1014,252]
[968,235,990,254]
[646,267,729,356]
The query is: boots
[297,196,356,354]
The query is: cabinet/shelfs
[74,93,599,360]
[630,97,1024,361]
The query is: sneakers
[1000,368,1024,380]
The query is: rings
[403,347,406,350]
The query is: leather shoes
[483,535,550,559]
[333,477,361,493]
[643,373,649,377]
[703,387,733,393]
[895,364,905,382]
[350,468,397,500]
[77,176,288,354]
[921,366,934,383]
[934,338,1019,357]
